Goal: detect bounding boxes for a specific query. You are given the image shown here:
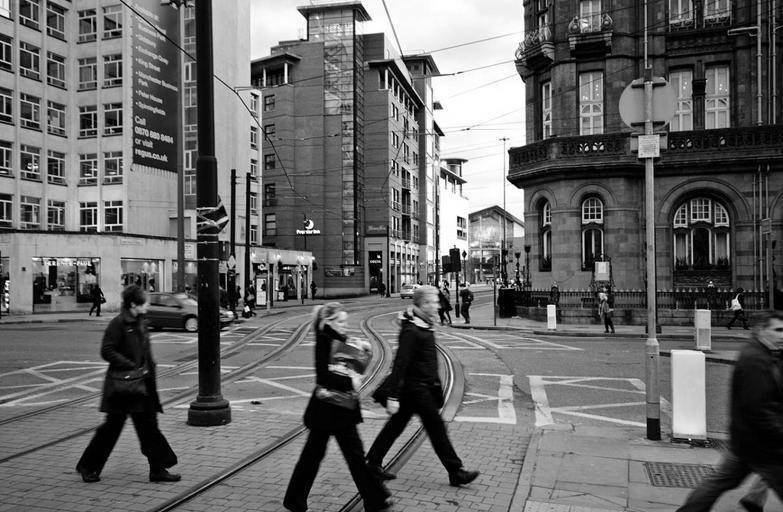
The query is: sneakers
[739,499,764,512]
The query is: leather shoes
[149,471,180,482]
[76,466,100,483]
[364,500,393,512]
[449,468,478,485]
[373,470,396,481]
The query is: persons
[675,310,783,512]
[437,279,474,325]
[365,285,480,487]
[727,287,751,330]
[283,302,394,512]
[76,284,182,483]
[740,474,770,512]
[378,280,386,297]
[602,284,615,333]
[89,284,104,316]
[236,274,318,318]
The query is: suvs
[400,284,421,299]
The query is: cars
[145,293,232,331]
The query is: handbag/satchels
[372,374,398,408]
[315,339,373,409]
[102,365,149,402]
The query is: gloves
[386,397,401,415]
